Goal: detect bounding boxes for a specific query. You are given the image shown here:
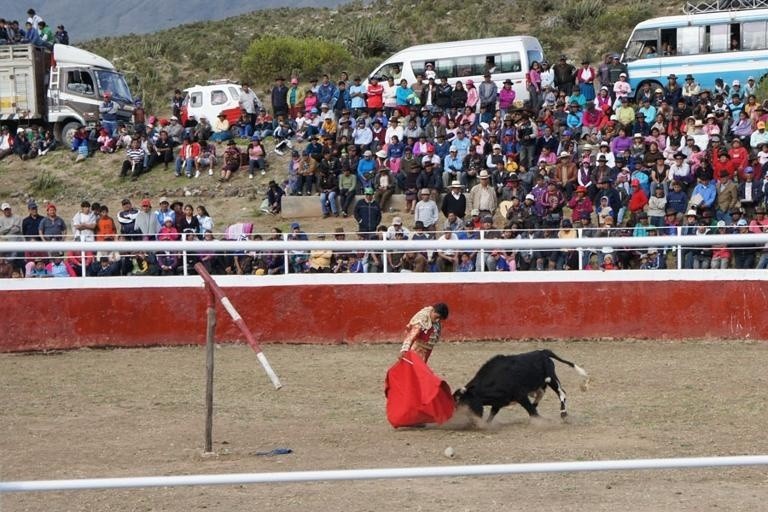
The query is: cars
[176,77,265,131]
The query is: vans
[354,35,547,114]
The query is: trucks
[0,41,136,152]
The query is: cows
[451,347,588,423]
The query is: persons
[399,303,449,429]
[0,8,768,278]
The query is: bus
[612,6,767,101]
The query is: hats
[556,100,595,109]
[310,103,327,114]
[420,189,430,195]
[465,209,492,227]
[122,196,183,222]
[667,207,713,218]
[418,107,515,152]
[684,113,715,126]
[512,194,535,201]
[364,187,374,195]
[667,74,694,80]
[448,180,464,187]
[347,145,387,158]
[620,73,626,77]
[732,76,755,86]
[654,152,686,161]
[558,141,623,163]
[542,54,566,65]
[1,203,10,211]
[505,175,522,182]
[563,177,639,227]
[633,113,646,139]
[410,161,434,169]
[477,171,489,179]
[754,105,766,130]
[717,207,765,226]
[28,203,36,208]
[391,217,425,229]
[699,170,728,180]
[227,140,236,145]
[466,72,513,85]
[339,108,400,125]
[250,136,259,142]
[275,76,298,84]
